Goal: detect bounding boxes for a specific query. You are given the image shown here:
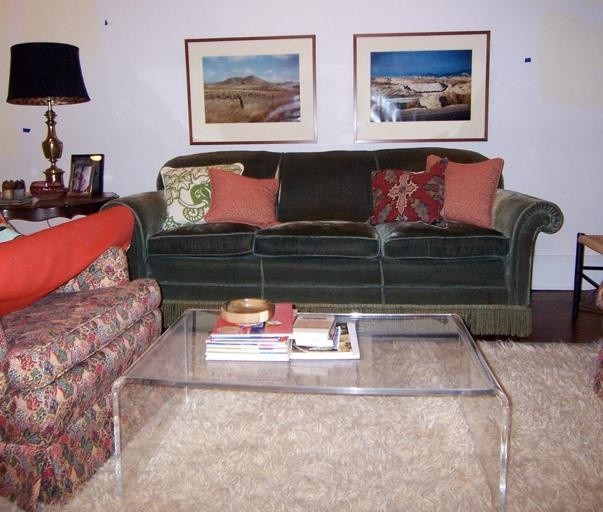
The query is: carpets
[0,337,603,512]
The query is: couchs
[97,147,566,341]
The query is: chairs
[0,245,163,507]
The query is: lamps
[5,41,90,184]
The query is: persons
[72,171,89,193]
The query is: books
[205,303,360,362]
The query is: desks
[0,191,118,222]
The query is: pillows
[370,152,505,232]
[156,161,281,232]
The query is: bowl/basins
[30,180,68,201]
[219,298,276,324]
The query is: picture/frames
[183,34,318,146]
[351,30,492,145]
[65,153,104,200]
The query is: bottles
[2,178,25,199]
